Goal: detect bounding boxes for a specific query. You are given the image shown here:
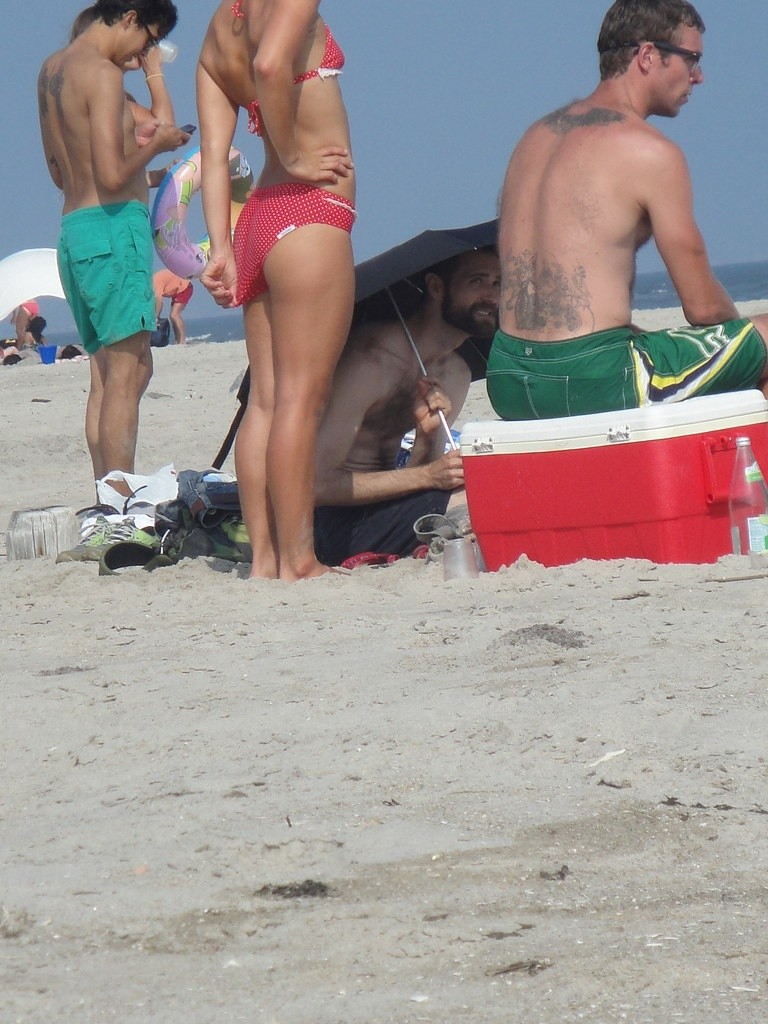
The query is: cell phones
[178,124,197,133]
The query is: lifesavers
[149,144,254,283]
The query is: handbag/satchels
[150,318,171,347]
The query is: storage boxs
[459,388,768,574]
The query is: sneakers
[56,515,159,564]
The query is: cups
[158,38,179,63]
[443,538,479,581]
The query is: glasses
[124,9,159,49]
[652,42,703,72]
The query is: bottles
[728,437,768,556]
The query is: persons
[38,0,191,510]
[0,298,90,365]
[311,248,500,570]
[195,1,357,579]
[153,268,193,344]
[487,0,768,419]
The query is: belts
[191,492,239,523]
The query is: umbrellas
[236,218,503,450]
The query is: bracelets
[146,73,164,82]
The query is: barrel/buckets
[38,345,57,364]
